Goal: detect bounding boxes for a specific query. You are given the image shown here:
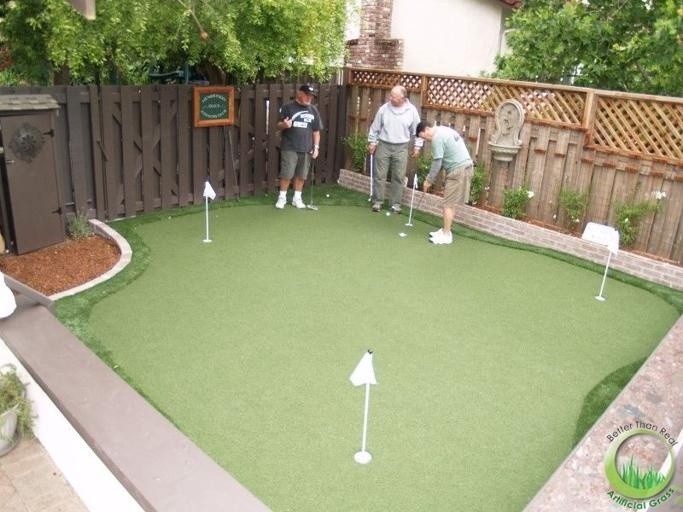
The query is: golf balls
[385,212,390,216]
[326,194,329,197]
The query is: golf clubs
[306,160,317,210]
[368,153,373,202]
[409,192,425,224]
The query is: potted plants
[0,361,41,452]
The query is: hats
[299,85,316,96]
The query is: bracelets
[314,144,320,149]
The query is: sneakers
[275,195,287,209]
[428,228,453,244]
[391,204,401,213]
[291,197,306,209]
[372,203,382,212]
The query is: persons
[367,85,424,213]
[416,121,473,244]
[276,85,323,209]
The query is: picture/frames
[192,85,235,128]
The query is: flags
[608,230,619,255]
[349,352,377,386]
[413,175,418,189]
[203,181,216,200]
